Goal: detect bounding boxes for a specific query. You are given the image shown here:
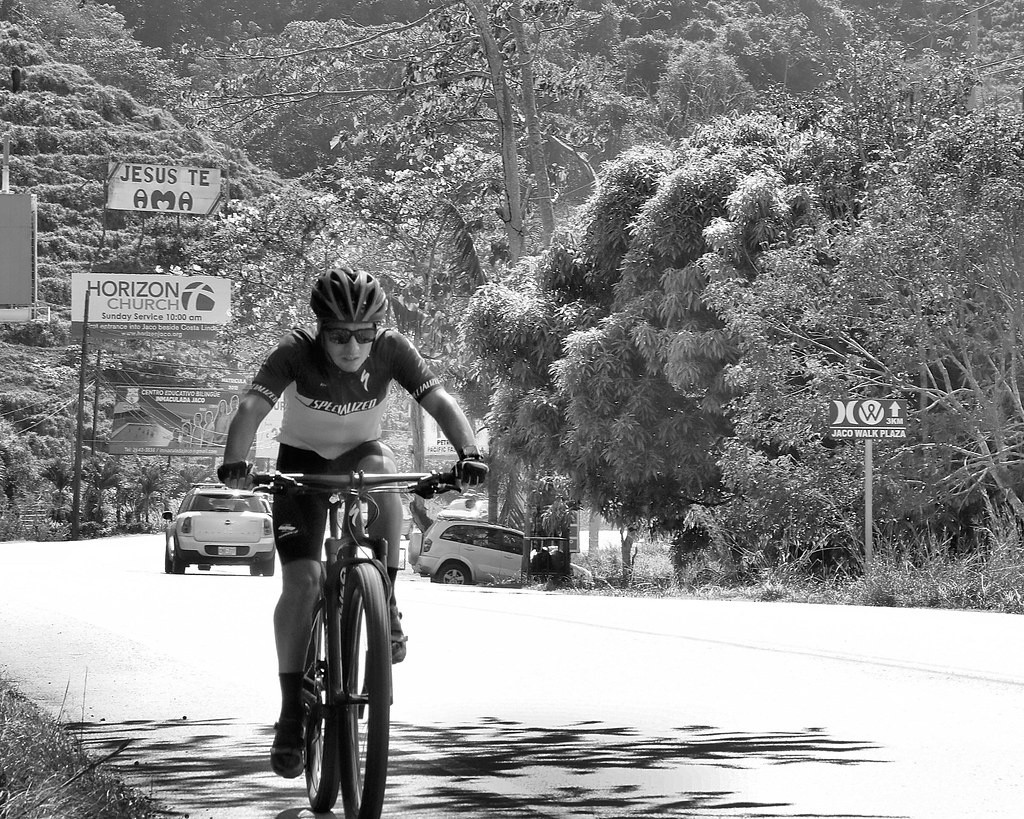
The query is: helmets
[309,268,389,325]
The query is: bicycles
[236,468,465,819]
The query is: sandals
[390,606,408,664]
[270,717,305,779]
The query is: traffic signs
[830,398,909,441]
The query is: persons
[221,267,490,780]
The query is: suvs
[407,517,595,591]
[160,483,275,577]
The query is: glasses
[320,326,376,344]
